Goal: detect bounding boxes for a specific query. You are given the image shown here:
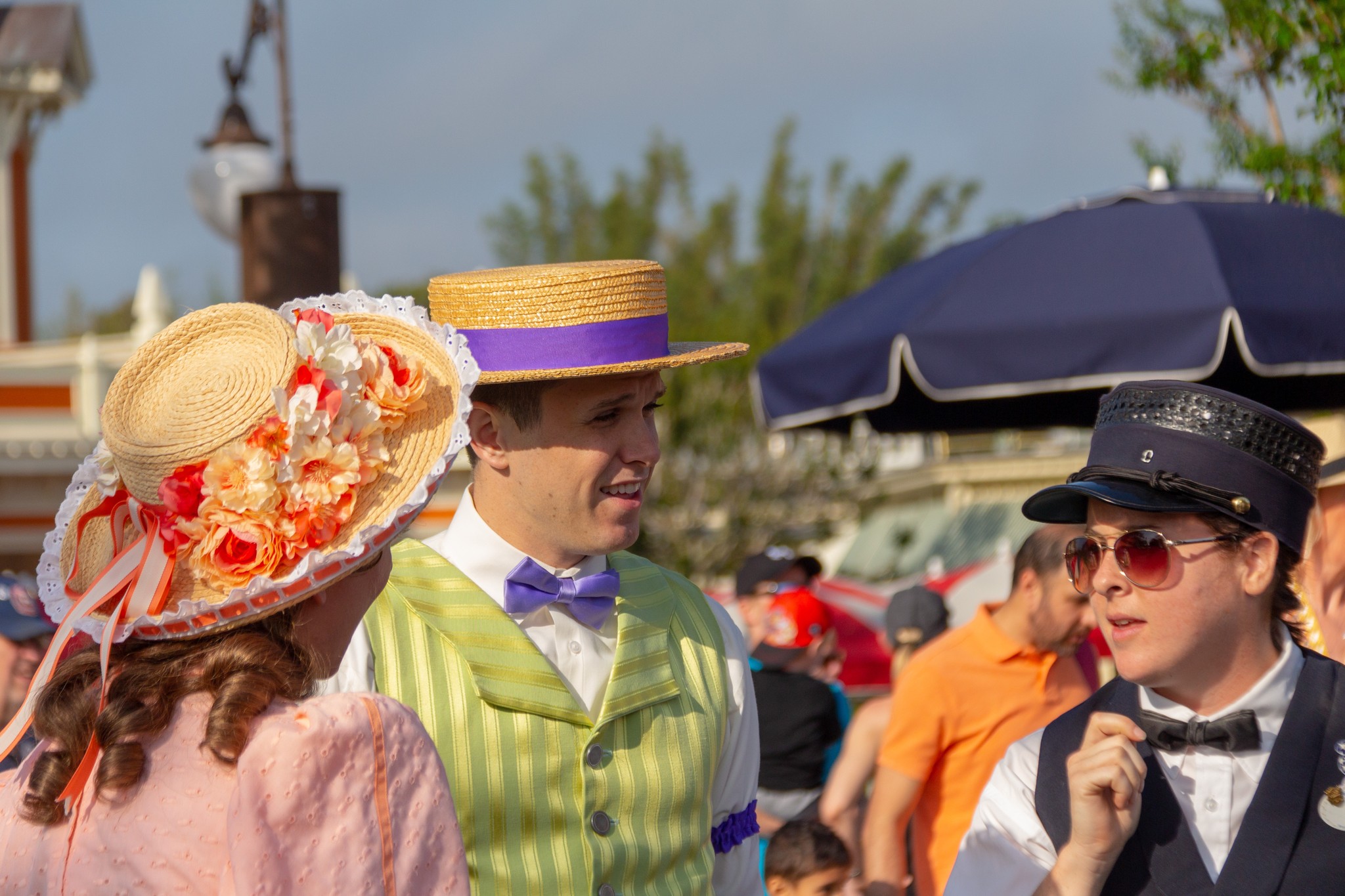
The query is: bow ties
[1137,708,1263,752]
[504,557,621,631]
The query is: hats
[763,587,833,649]
[1021,380,1328,564]
[0,574,56,643]
[0,288,482,805]
[426,258,751,386]
[1300,414,1345,486]
[885,584,949,646]
[734,546,823,599]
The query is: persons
[0,292,473,896]
[312,259,766,896]
[0,569,60,768]
[946,380,1345,896]
[705,527,1096,896]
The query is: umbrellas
[751,172,1345,430]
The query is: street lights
[202,0,344,304]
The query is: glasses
[820,650,841,668]
[755,582,794,597]
[1063,532,1249,596]
